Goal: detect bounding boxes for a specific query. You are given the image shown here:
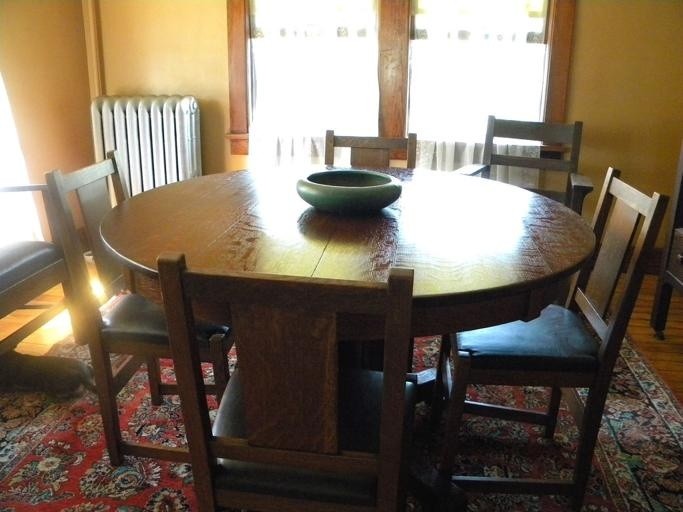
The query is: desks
[100,168,598,511]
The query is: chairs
[153,250,416,511]
[325,130,416,169]
[651,151,683,332]
[421,165,669,512]
[456,115,594,214]
[1,188,87,355]
[44,150,238,466]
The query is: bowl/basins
[296,169,403,216]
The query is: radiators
[90,96,205,202]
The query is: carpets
[1,290,683,509]
